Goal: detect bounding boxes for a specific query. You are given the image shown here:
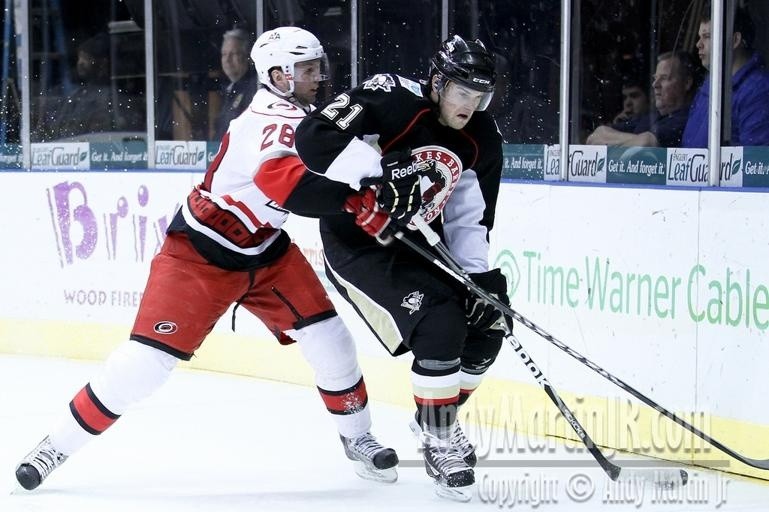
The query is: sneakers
[419,434,477,488]
[11,430,72,492]
[415,410,478,472]
[339,432,401,471]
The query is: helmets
[249,25,326,97]
[424,29,498,112]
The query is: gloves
[348,190,405,246]
[463,267,515,341]
[374,144,424,222]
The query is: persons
[211,28,262,145]
[294,35,515,490]
[678,5,768,151]
[27,30,142,143]
[608,72,652,128]
[13,24,405,494]
[587,49,702,148]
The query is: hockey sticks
[408,209,688,492]
